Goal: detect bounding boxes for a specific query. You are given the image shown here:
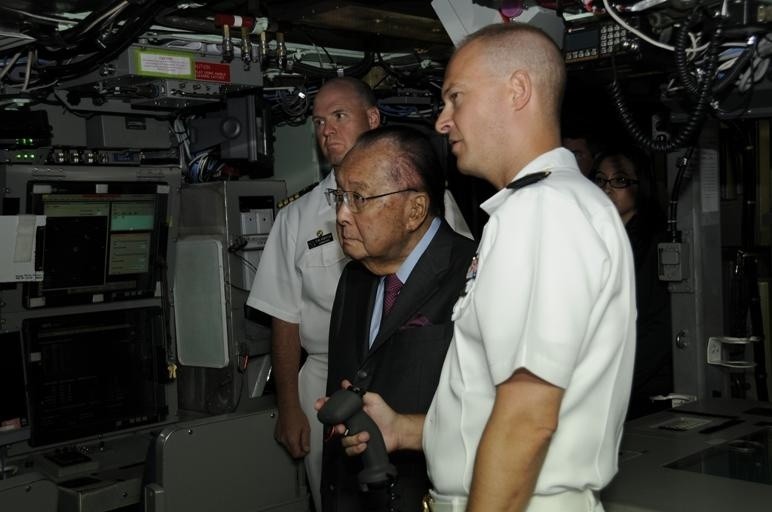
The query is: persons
[320,126,478,511]
[314,23,638,512]
[563,119,670,420]
[246,78,476,512]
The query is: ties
[381,273,402,324]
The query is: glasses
[593,176,638,189]
[323,189,418,209]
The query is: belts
[422,488,608,511]
